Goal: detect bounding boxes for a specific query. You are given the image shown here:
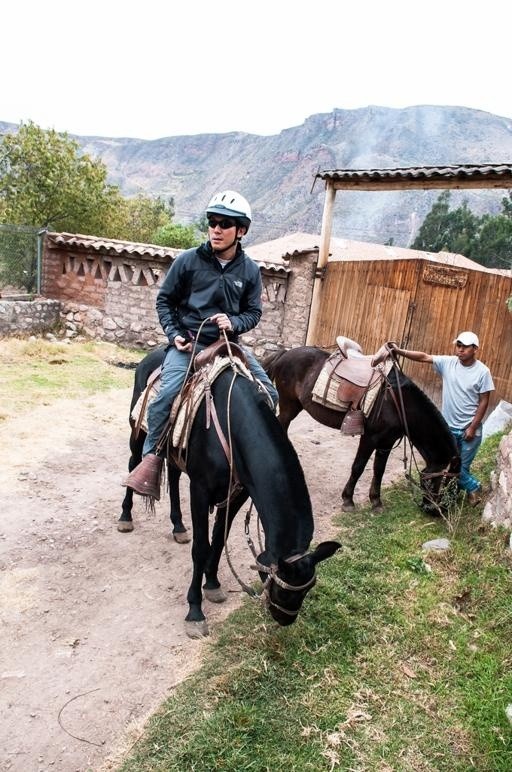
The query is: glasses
[207,217,238,230]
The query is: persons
[117,188,282,501]
[388,330,496,508]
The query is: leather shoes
[468,481,484,506]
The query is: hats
[452,331,480,350]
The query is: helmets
[205,189,252,236]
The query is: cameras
[181,330,195,346]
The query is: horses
[260,345,462,517]
[117,346,343,641]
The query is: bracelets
[404,350,408,356]
